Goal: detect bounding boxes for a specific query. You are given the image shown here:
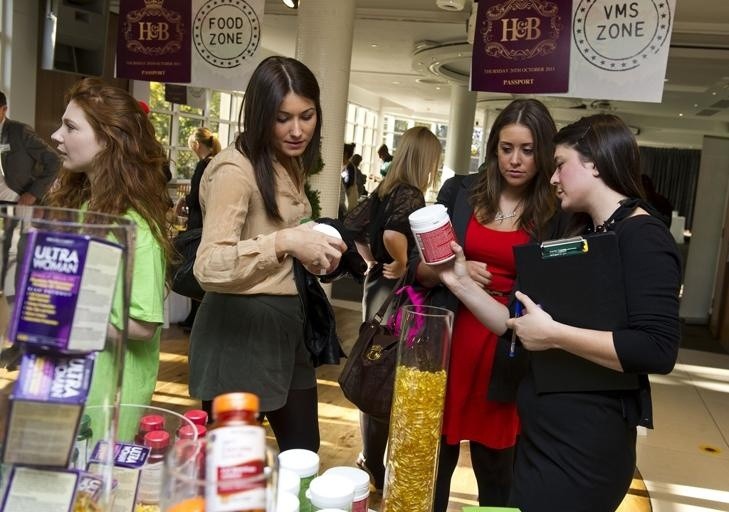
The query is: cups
[0,201,210,510]
[383,303,455,510]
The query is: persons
[0,90,63,330]
[431,99,558,511]
[174,127,221,326]
[369,144,394,183]
[343,126,442,489]
[350,154,368,202]
[36,78,173,462]
[340,143,358,215]
[188,55,347,454]
[430,113,683,512]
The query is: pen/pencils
[509,299,522,358]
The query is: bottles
[304,225,342,277]
[409,205,460,265]
[276,447,371,511]
[204,392,268,512]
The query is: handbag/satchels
[338,317,402,416]
